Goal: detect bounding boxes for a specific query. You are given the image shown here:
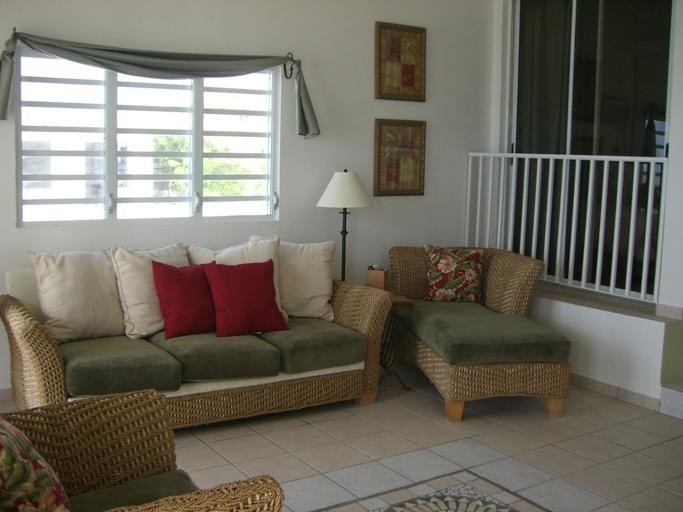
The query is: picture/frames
[373,21,427,102]
[372,118,427,197]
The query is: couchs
[0,387,284,511]
[0,268,393,431]
[388,245,573,423]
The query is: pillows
[152,261,217,339]
[424,246,485,303]
[110,242,192,339]
[187,236,288,323]
[202,258,291,338]
[30,249,125,345]
[249,239,335,322]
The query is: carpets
[309,468,550,511]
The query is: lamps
[314,168,372,280]
